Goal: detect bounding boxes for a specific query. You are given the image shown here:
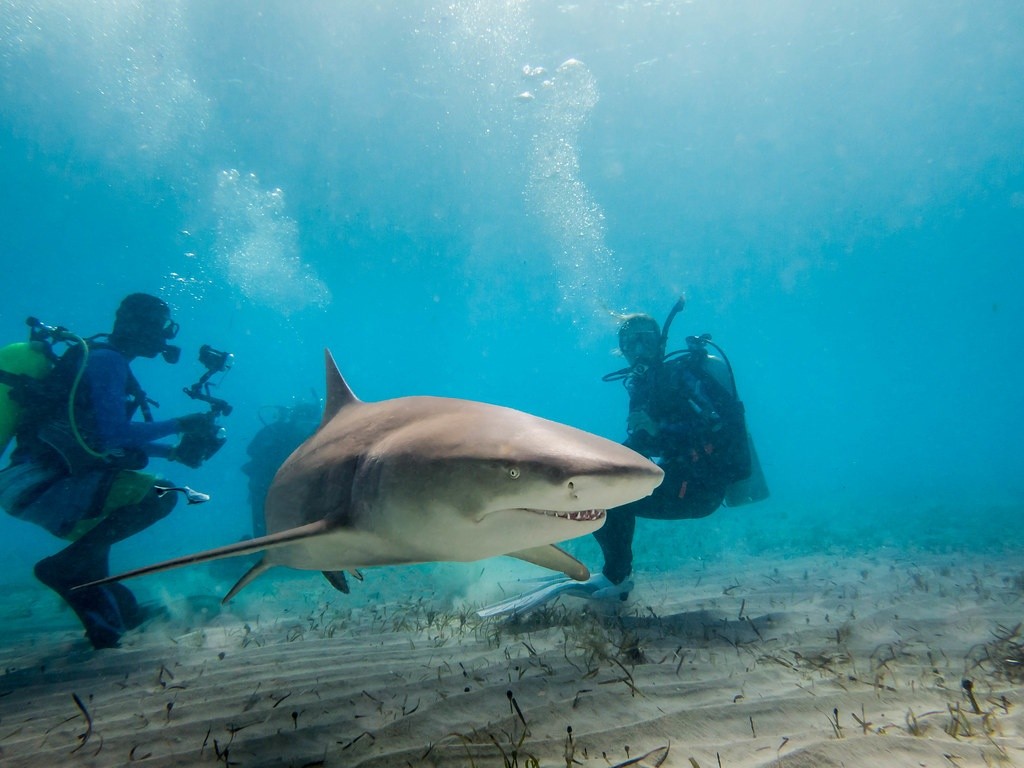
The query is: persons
[212,401,323,579]
[472,315,749,620]
[0,291,219,646]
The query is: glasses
[156,315,179,340]
[619,330,660,353]
